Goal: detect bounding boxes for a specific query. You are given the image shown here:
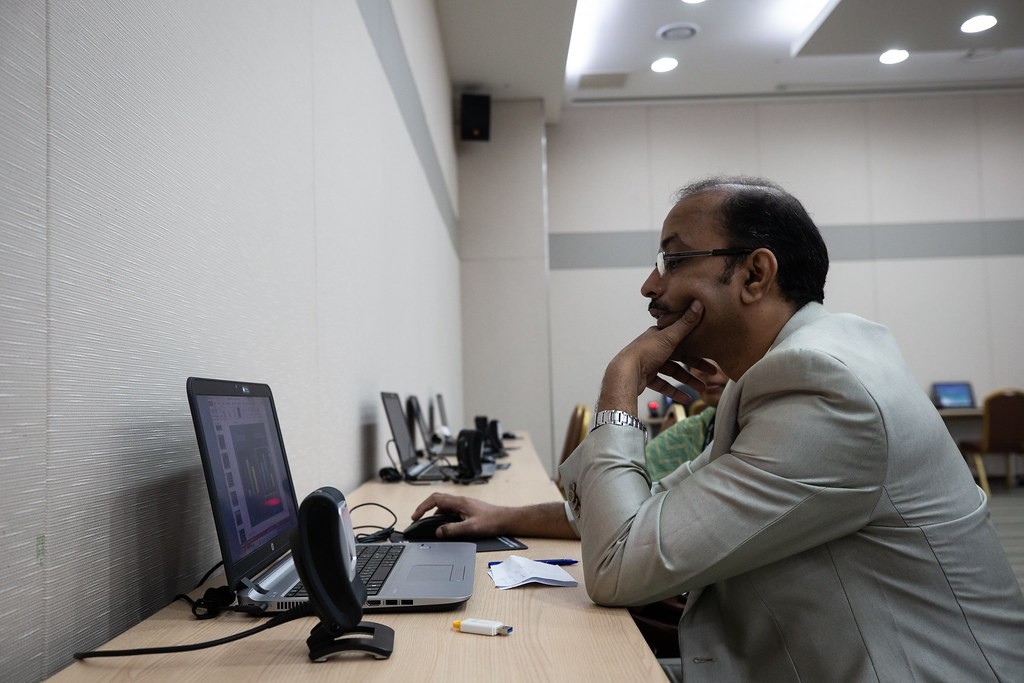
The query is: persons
[411,176,1024,683]
[645,358,729,483]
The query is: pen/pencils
[488,558,581,568]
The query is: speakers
[288,485,395,663]
[461,94,491,141]
[457,413,507,484]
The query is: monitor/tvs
[934,383,974,409]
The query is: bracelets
[594,410,648,446]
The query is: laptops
[185,378,504,615]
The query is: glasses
[652,245,761,279]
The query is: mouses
[403,514,464,541]
